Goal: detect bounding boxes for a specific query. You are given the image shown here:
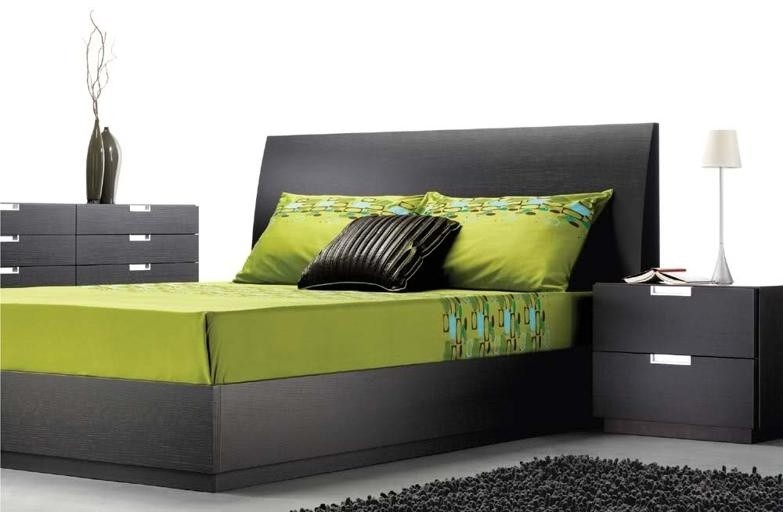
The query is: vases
[101,126,119,204]
[85,119,105,204]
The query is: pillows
[297,216,462,292]
[414,189,614,293]
[232,192,424,283]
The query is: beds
[0,123,658,494]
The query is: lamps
[701,129,742,284]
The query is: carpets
[288,454,782,512]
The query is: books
[623,267,684,284]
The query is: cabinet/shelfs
[0,204,199,286]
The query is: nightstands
[590,282,781,445]
